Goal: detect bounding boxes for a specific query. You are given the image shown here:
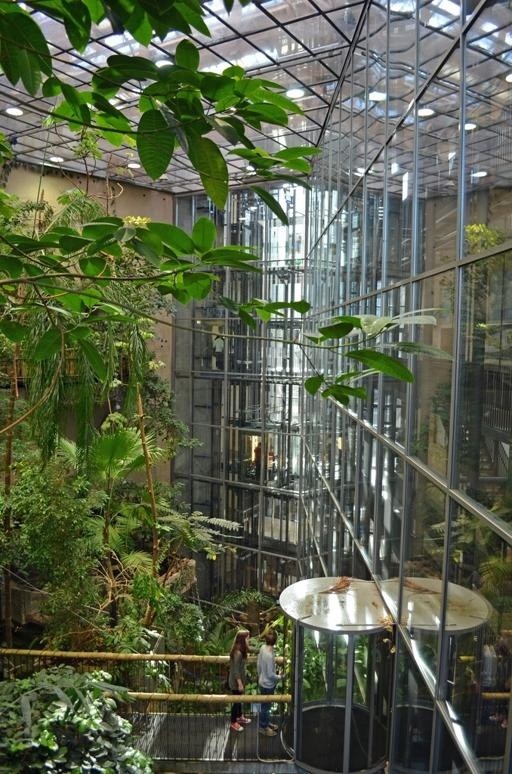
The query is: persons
[226,628,253,732]
[256,629,288,737]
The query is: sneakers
[259,726,277,736]
[268,722,278,730]
[237,718,252,724]
[231,723,244,732]
